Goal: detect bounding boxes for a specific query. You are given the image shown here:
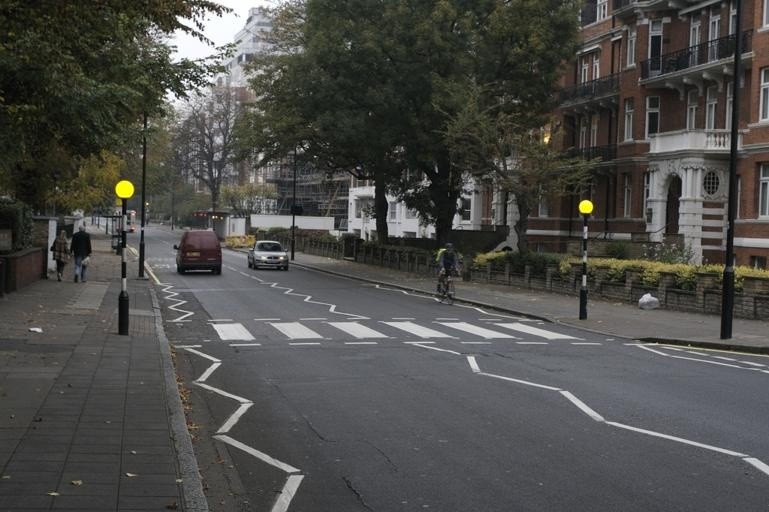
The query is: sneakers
[56,271,89,284]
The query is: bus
[115,209,137,233]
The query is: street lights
[576,198,595,320]
[115,179,136,336]
[114,199,124,255]
[138,103,169,280]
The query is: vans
[173,227,222,276]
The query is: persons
[435,242,460,293]
[70,225,92,283]
[52,229,73,282]
[145,212,150,225]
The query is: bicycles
[432,267,464,306]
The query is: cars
[247,239,290,272]
